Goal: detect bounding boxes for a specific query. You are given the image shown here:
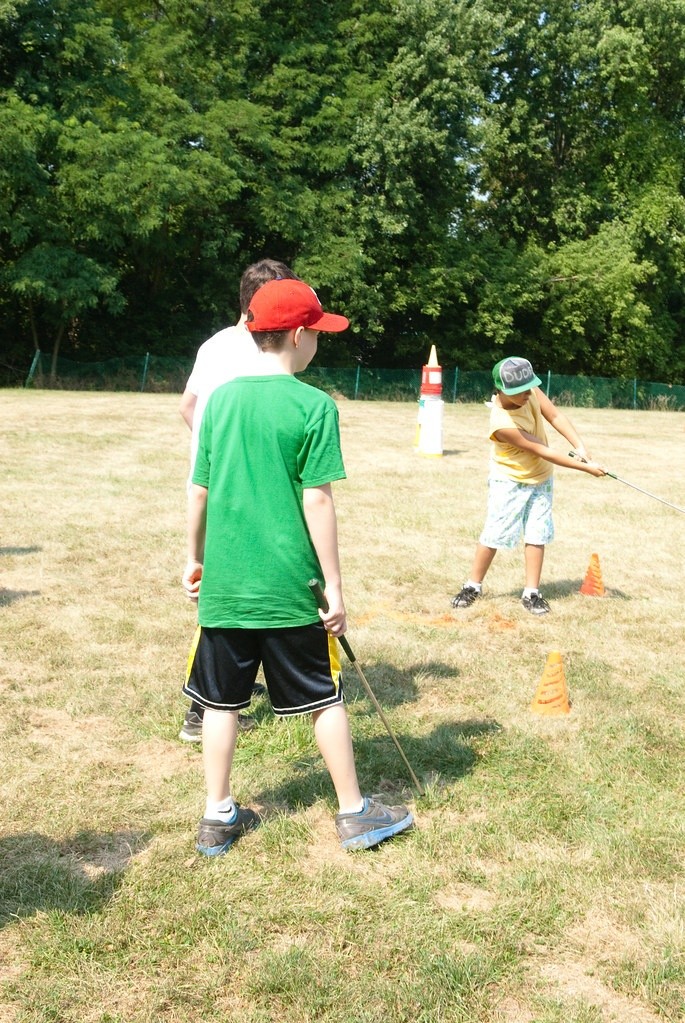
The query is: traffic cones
[580,553,604,596]
[531,649,573,714]
[424,345,439,368]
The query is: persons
[179,256,305,741]
[452,354,605,615]
[189,277,414,853]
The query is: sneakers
[335,796,414,856]
[180,709,257,743]
[452,583,478,611]
[520,592,550,615]
[246,681,266,694]
[195,801,264,859]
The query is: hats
[494,354,542,396]
[244,277,351,338]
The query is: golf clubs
[305,575,435,813]
[564,449,685,520]
